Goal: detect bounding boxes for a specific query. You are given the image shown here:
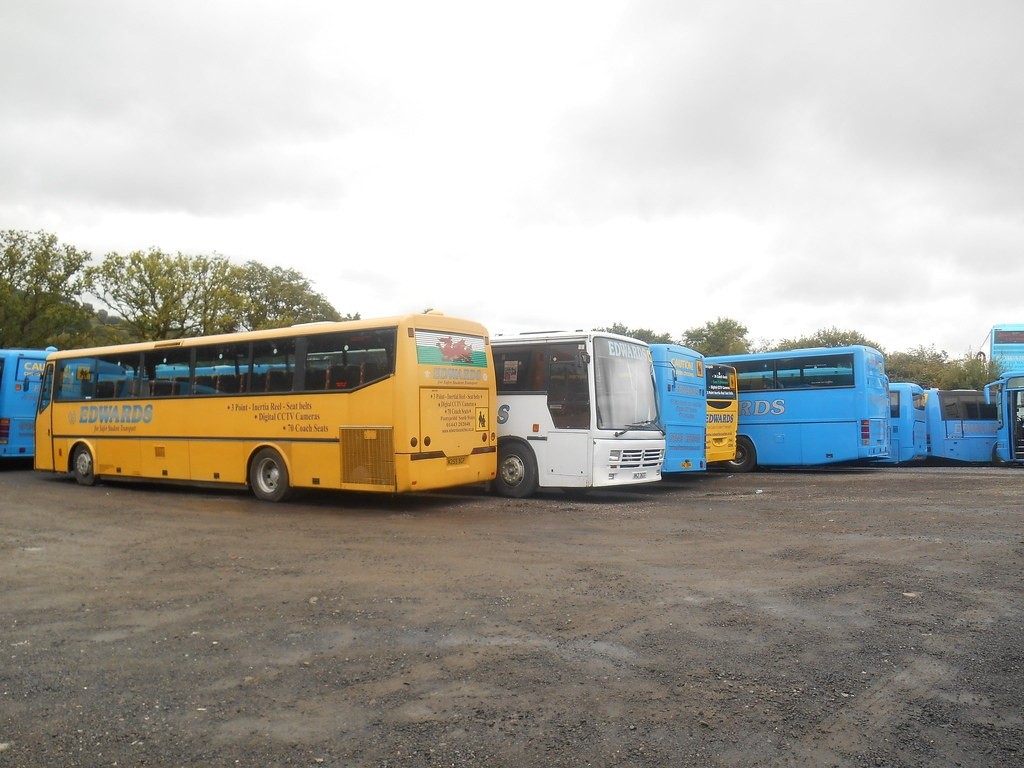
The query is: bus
[706,364,738,464]
[704,344,892,473]
[984,370,1024,462]
[922,389,1013,467]
[849,382,928,465]
[23,311,497,502]
[96,364,296,398]
[306,329,678,500]
[0,346,93,458]
[548,344,708,473]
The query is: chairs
[95,362,382,400]
